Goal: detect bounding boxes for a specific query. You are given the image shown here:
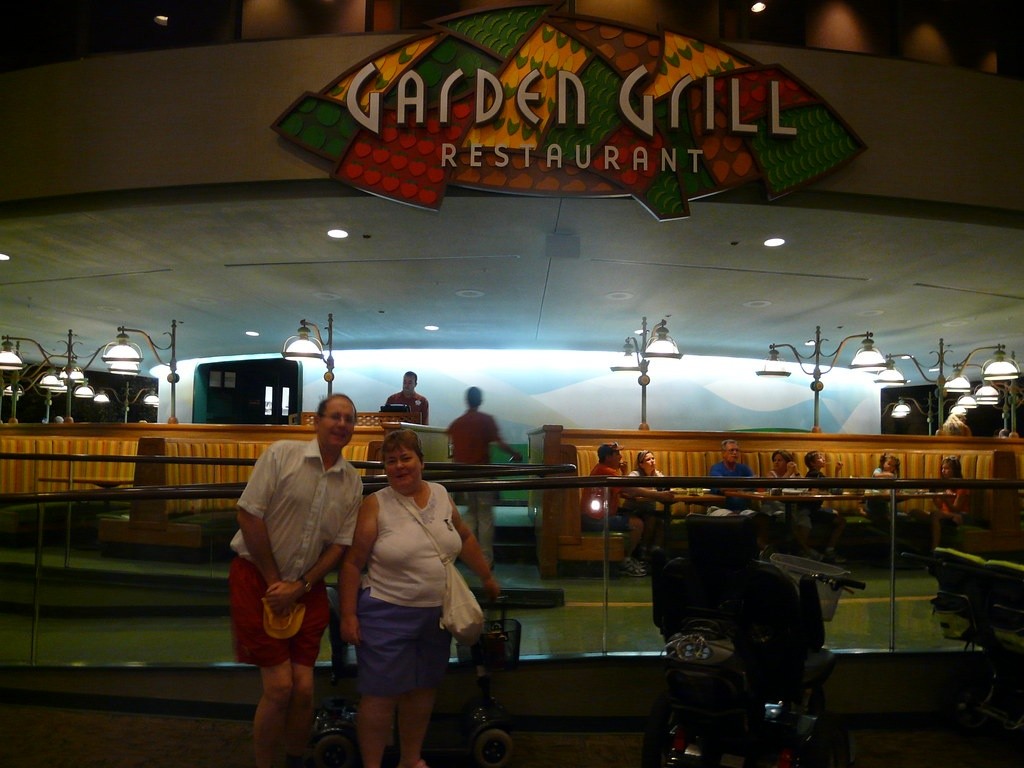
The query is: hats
[260,594,305,640]
[597,440,627,460]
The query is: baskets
[471,617,522,669]
[770,553,850,621]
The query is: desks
[40,473,139,512]
[624,491,730,560]
[724,490,957,563]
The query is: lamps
[756,326,1024,436]
[0,319,183,425]
[283,313,336,396]
[608,317,683,429]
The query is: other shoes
[827,549,849,564]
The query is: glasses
[319,410,357,426]
[723,448,741,454]
[943,456,958,463]
[611,442,618,449]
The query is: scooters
[653,554,872,768]
[303,586,522,768]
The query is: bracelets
[795,473,799,476]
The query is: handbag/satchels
[442,560,486,644]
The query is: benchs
[536,443,1015,571]
[1,435,393,566]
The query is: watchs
[300,577,311,592]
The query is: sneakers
[619,558,650,578]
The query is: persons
[341,430,499,768]
[623,450,664,559]
[580,442,648,577]
[855,455,900,518]
[447,387,522,570]
[763,452,802,553]
[229,395,364,767]
[383,372,428,425]
[706,441,774,552]
[908,455,971,551]
[936,414,1019,438]
[803,451,846,562]
